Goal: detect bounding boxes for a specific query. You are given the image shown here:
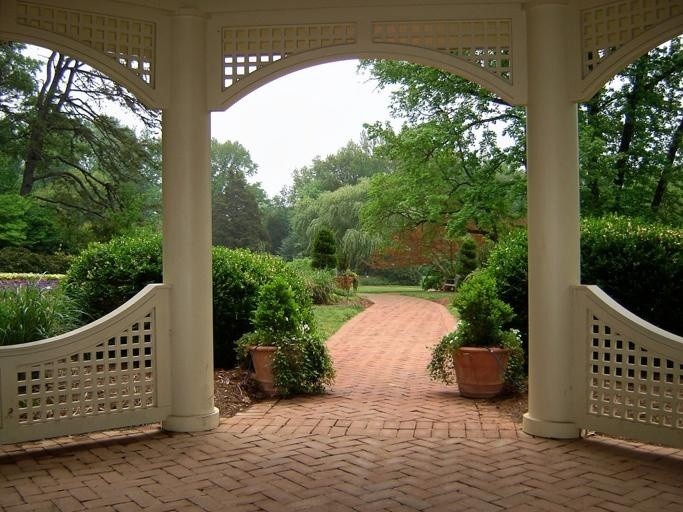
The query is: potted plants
[442,265,456,292]
[426,267,525,400]
[234,277,337,397]
[335,270,359,297]
